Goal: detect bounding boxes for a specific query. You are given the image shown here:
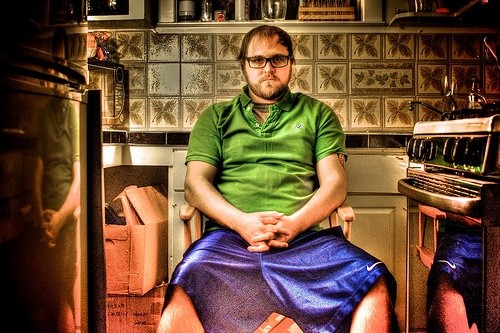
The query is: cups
[214,10,226,22]
[261,0,287,22]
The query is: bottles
[178,0,196,23]
[201,0,212,22]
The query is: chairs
[178,203,356,333]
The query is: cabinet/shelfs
[87,0,500,35]
[167,150,409,333]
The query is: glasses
[244,54,291,69]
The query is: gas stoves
[406,102,500,176]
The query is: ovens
[397,166,500,333]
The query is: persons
[157,24,397,333]
[16,93,81,332]
[426,213,484,333]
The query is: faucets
[407,99,450,122]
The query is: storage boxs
[104,220,168,296]
[105,283,168,333]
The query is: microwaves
[87,58,124,126]
